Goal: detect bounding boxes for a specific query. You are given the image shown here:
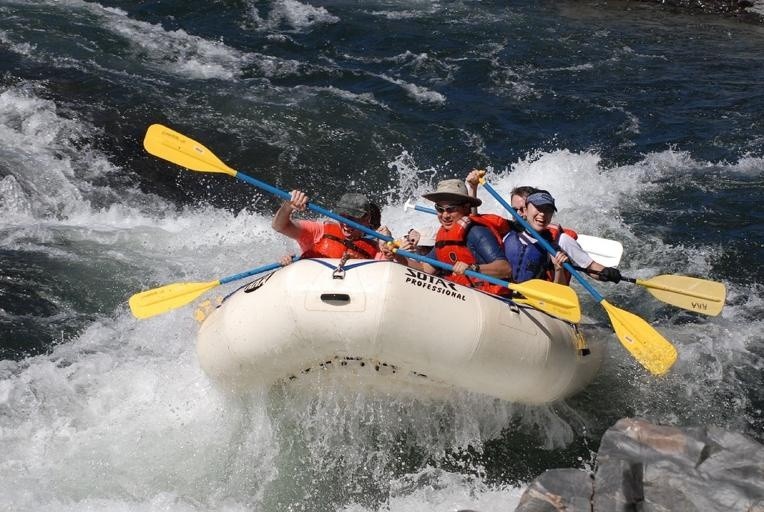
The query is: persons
[465,170,567,299]
[279,203,421,266]
[271,189,399,263]
[399,178,513,299]
[508,184,621,284]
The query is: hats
[337,192,372,219]
[525,192,558,210]
[421,179,481,205]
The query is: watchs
[470,263,480,273]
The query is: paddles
[402,198,624,270]
[572,266,727,319]
[473,170,678,378]
[124,255,303,322]
[383,241,583,326]
[192,295,230,322]
[143,123,395,244]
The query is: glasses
[433,203,461,212]
[511,205,525,213]
